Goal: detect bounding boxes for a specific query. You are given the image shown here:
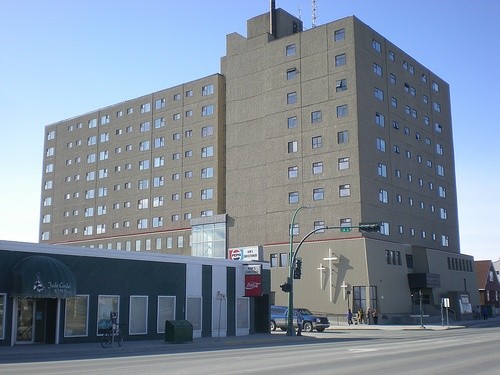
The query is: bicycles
[101,327,124,347]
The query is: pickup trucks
[270,305,330,332]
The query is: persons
[366,307,371,325]
[475,305,481,320]
[347,309,352,325]
[482,306,489,320]
[371,307,378,325]
[297,309,302,336]
[358,308,363,324]
